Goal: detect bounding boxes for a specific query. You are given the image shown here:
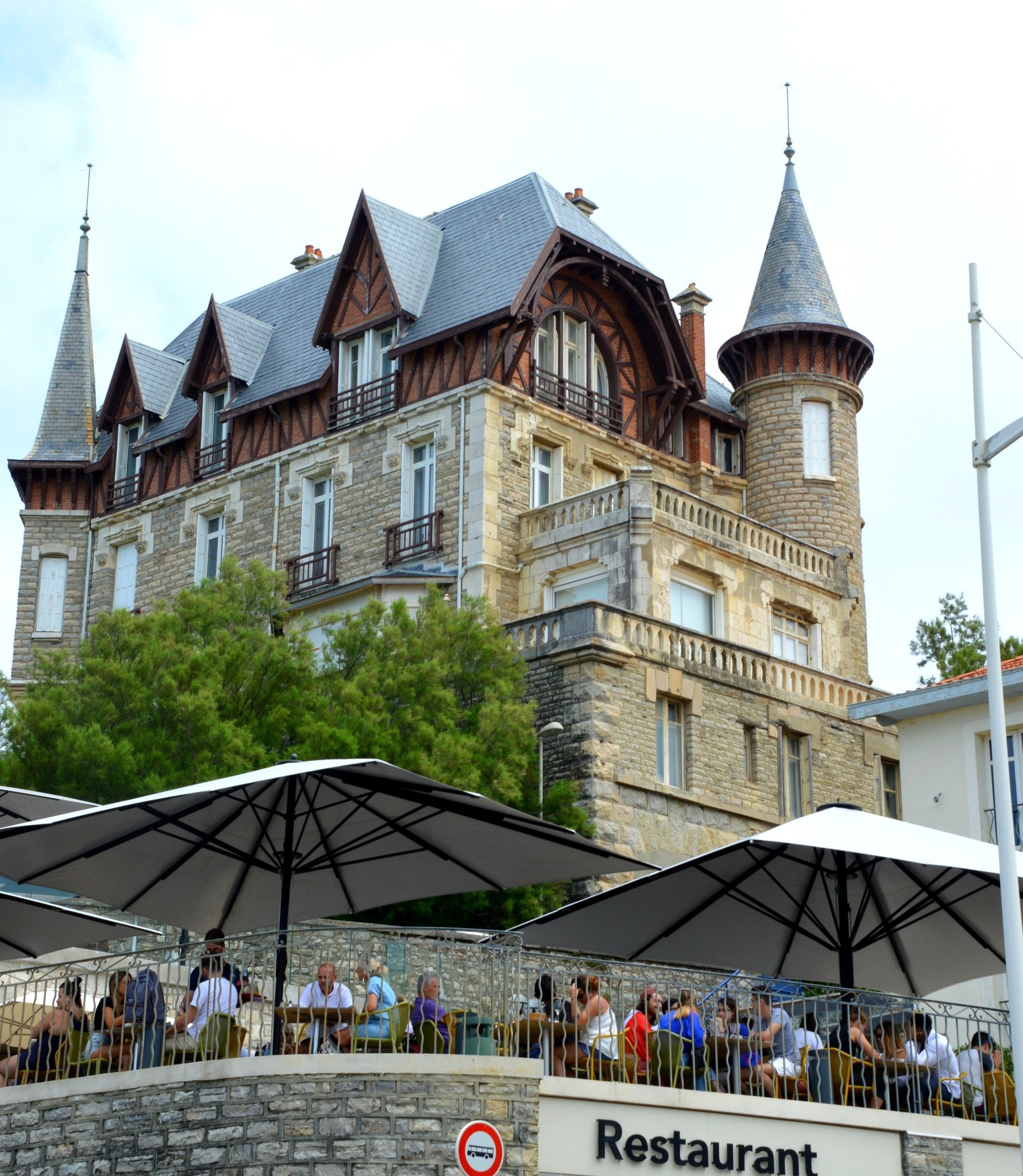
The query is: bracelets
[570,997,575,1001]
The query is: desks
[275,1007,357,1054]
[706,1036,778,1094]
[495,1019,592,1076]
[866,1058,938,1111]
[94,1024,142,1070]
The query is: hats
[640,988,656,1002]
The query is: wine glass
[554,1000,562,1022]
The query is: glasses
[364,953,368,966]
[876,1033,882,1039]
[577,991,586,1000]
[119,979,132,985]
[281,1032,289,1038]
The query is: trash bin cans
[461,1017,498,1055]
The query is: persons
[163,927,252,1064]
[623,982,796,1099]
[522,973,619,1078]
[84,971,139,1072]
[795,1008,1003,1118]
[410,969,453,1054]
[296,953,402,1054]
[0,973,90,1089]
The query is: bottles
[254,981,259,995]
[279,1001,285,1007]
[181,1001,184,1012]
[232,963,237,985]
[288,1001,292,1007]
[242,963,249,987]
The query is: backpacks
[127,966,165,1024]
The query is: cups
[730,1023,740,1039]
[710,1020,719,1036]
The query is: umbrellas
[0,753,663,962]
[476,797,1023,1003]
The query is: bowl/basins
[529,1013,546,1021]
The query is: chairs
[22,1001,1018,1126]
